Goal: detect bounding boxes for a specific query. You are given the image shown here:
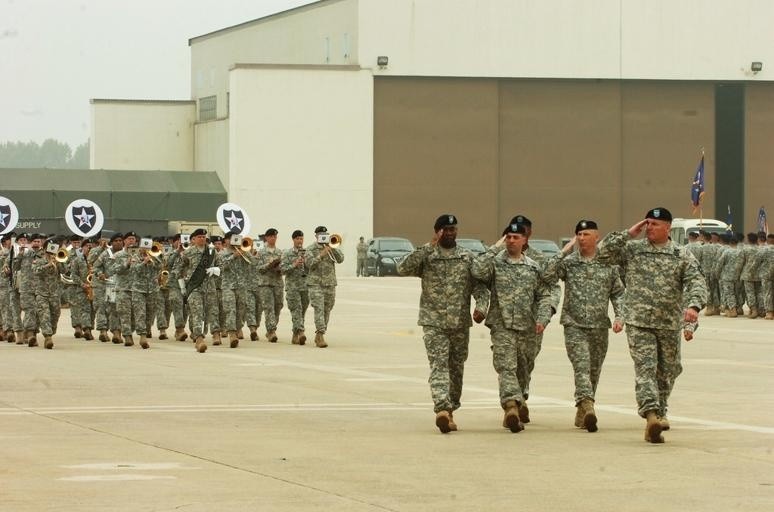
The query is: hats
[434,215,457,233]
[292,230,303,239]
[0,229,102,247]
[502,215,531,236]
[259,235,267,243]
[264,229,278,236]
[645,208,672,222]
[688,229,774,242]
[575,219,598,235]
[108,229,237,246]
[315,226,327,233]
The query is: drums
[10,243,29,290]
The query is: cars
[421,238,486,259]
[360,236,417,277]
[497,239,563,261]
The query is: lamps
[749,61,762,77]
[376,56,389,70]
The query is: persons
[598,207,708,442]
[683,229,774,320]
[540,219,629,432]
[491,214,562,422]
[356,236,369,277]
[470,223,552,433]
[0,226,344,354]
[397,214,490,432]
[677,318,702,385]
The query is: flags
[690,154,705,216]
[757,206,766,232]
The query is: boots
[0,325,55,349]
[503,401,530,432]
[241,325,327,347]
[161,325,238,352]
[575,398,597,432]
[436,411,457,433]
[645,411,670,443]
[704,305,774,320]
[73,324,150,350]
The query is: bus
[645,217,734,248]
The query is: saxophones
[158,261,170,288]
[86,251,94,300]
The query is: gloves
[204,266,221,278]
[178,278,187,296]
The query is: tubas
[49,248,69,275]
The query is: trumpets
[143,242,163,266]
[232,237,255,264]
[324,233,342,263]
